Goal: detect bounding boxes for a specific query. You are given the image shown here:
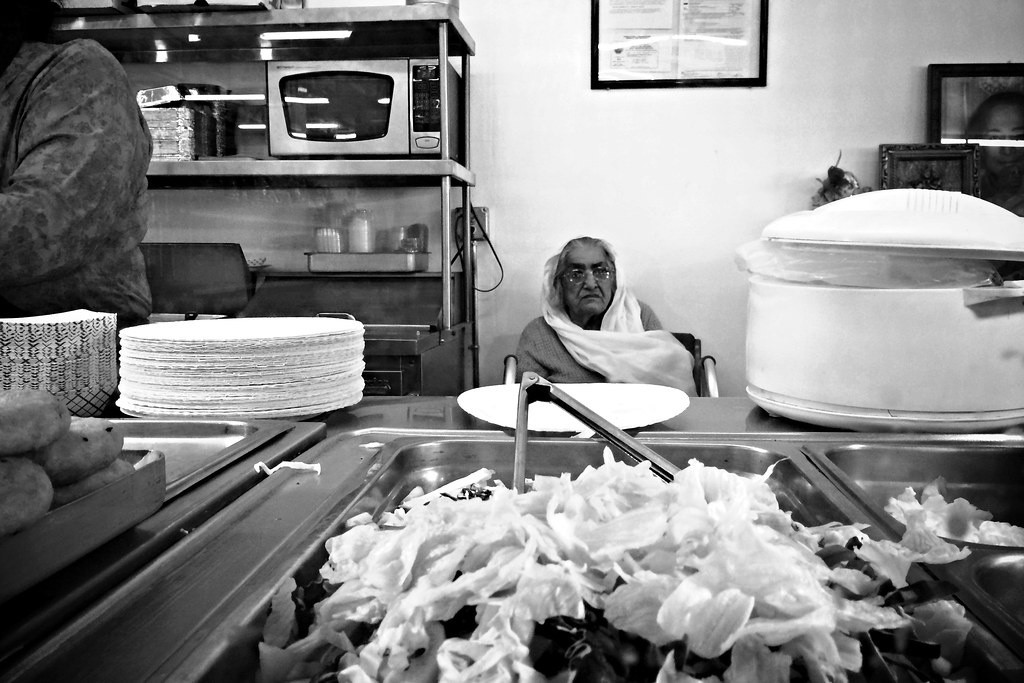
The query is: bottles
[350,209,375,254]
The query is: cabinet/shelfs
[0,3,481,398]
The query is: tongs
[513,372,680,494]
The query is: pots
[744,189,1024,433]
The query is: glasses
[560,266,614,283]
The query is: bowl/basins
[401,238,423,253]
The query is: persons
[0,0,152,330]
[962,92,1024,218]
[513,237,703,398]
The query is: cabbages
[261,446,1024,683]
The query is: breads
[0,390,136,533]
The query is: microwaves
[266,60,441,159]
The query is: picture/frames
[879,142,980,201]
[930,63,1024,216]
